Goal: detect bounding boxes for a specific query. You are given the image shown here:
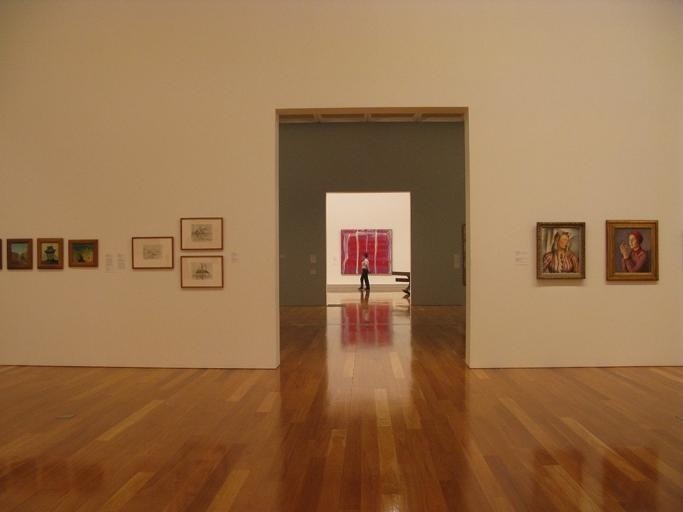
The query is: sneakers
[358,287,363,290]
[365,287,370,290]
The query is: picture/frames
[37,238,64,269]
[536,221,586,280]
[180,217,224,250]
[180,255,223,288]
[131,236,175,269]
[606,219,659,281]
[7,238,33,269]
[68,239,98,267]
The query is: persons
[542,231,578,273]
[43,246,59,265]
[620,231,648,272]
[358,252,371,290]
